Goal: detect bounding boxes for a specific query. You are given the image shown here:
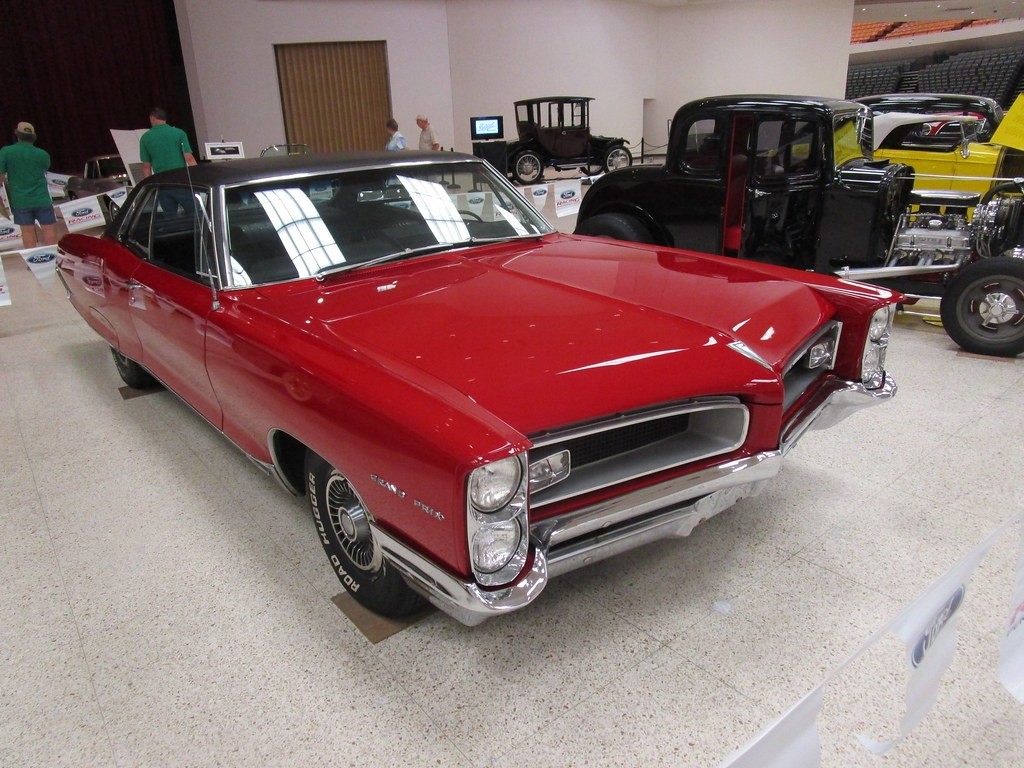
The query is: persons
[414,114,440,152]
[0,121,58,250]
[384,119,406,151]
[139,106,198,218]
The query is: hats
[414,113,429,121]
[17,122,35,135]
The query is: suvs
[504,95,634,185]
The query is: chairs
[154,224,289,283]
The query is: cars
[793,93,1005,152]
[55,149,906,630]
[64,155,134,225]
[572,93,1024,357]
[763,90,1024,219]
